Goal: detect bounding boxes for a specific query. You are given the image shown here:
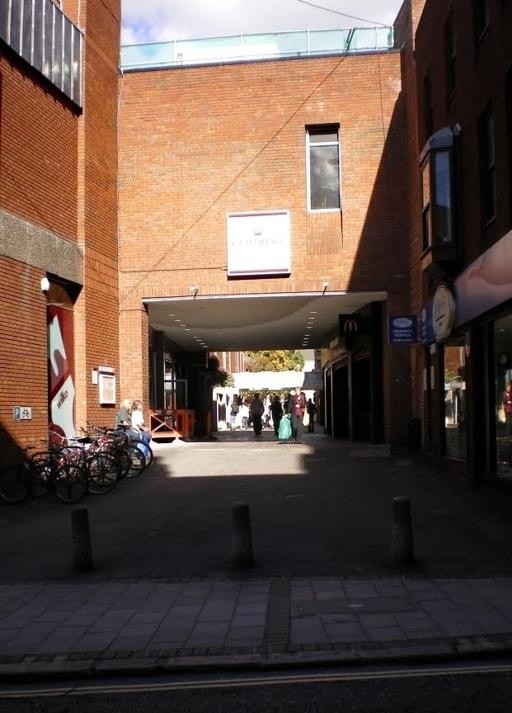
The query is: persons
[230,387,320,444]
[114,399,139,452]
[132,400,151,459]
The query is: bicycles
[0,423,154,504]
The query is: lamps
[40,277,50,291]
[322,283,329,296]
[191,287,199,300]
